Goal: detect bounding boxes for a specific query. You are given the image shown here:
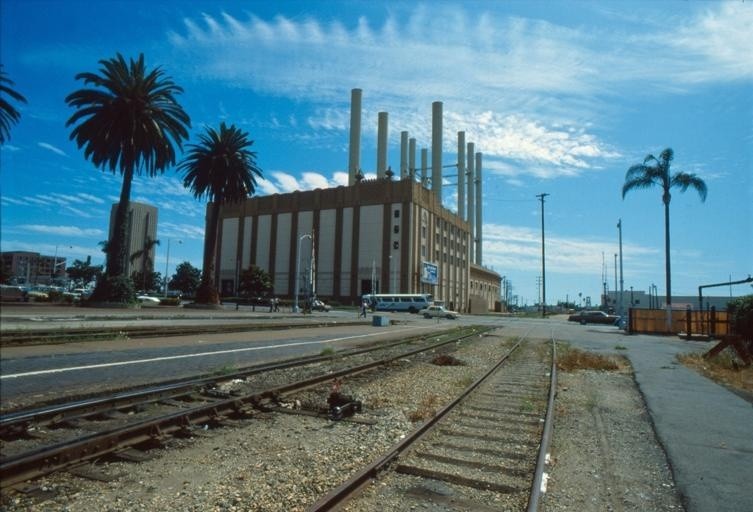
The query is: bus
[361,293,434,314]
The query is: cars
[418,306,462,319]
[312,299,331,313]
[167,290,187,300]
[0,280,96,302]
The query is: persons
[268,296,276,312]
[359,299,370,318]
[273,296,280,313]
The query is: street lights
[164,237,183,298]
[53,245,72,276]
[293,234,312,313]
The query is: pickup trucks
[568,311,621,325]
